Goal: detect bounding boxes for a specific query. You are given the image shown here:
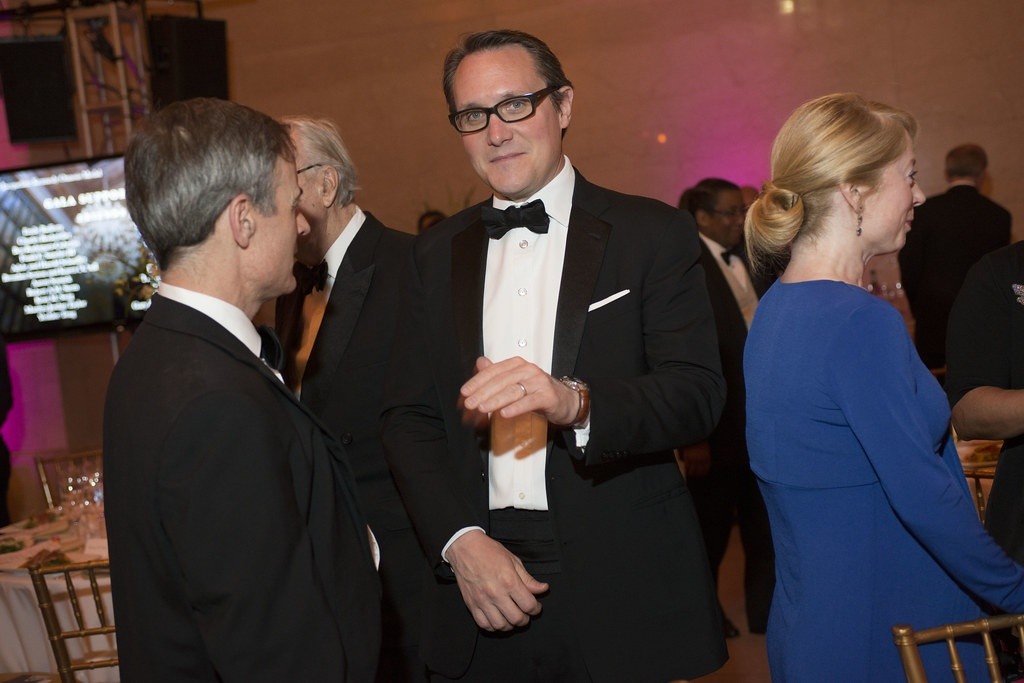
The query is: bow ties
[292,260,328,295]
[471,199,548,240]
[720,249,739,266]
[259,324,284,372]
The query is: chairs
[26,558,120,683]
[891,613,1024,683]
[34,448,103,508]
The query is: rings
[516,382,527,397]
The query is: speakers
[0,34,78,144]
[147,13,229,109]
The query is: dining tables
[0,519,121,683]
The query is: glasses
[448,85,560,133]
[712,207,747,217]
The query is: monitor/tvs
[0,152,163,341]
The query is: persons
[278,114,417,449]
[744,91,1024,683]
[687,177,760,400]
[101,98,377,683]
[740,186,758,215]
[376,28,729,683]
[901,141,1011,394]
[948,239,1024,573]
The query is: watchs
[560,375,589,430]
